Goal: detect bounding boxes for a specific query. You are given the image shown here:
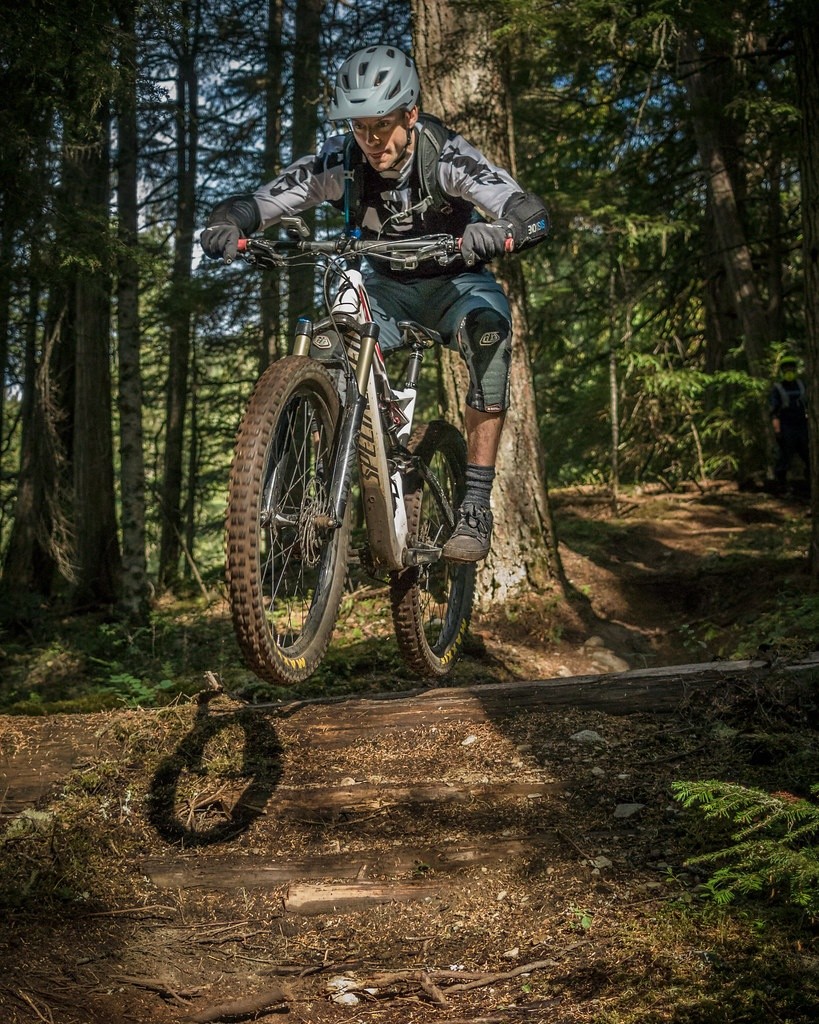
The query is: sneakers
[280,527,322,562]
[441,503,494,561]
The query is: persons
[765,355,811,501]
[200,46,550,562]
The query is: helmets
[328,44,420,120]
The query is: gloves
[460,220,515,267]
[202,217,249,264]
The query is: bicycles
[191,214,518,688]
